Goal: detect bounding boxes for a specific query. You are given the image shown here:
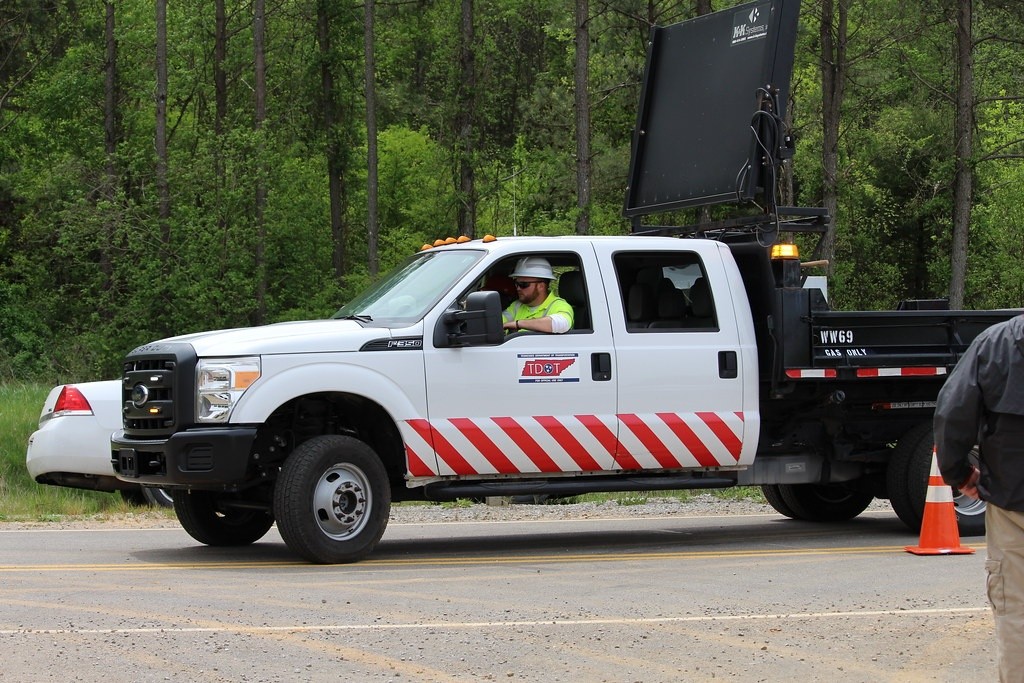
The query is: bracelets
[516,320,521,330]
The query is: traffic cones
[907,442,975,554]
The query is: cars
[24,380,173,509]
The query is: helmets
[508,257,557,280]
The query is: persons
[484,255,574,335]
[932,312,1024,683]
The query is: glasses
[514,278,542,288]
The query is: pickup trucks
[108,3,1022,564]
